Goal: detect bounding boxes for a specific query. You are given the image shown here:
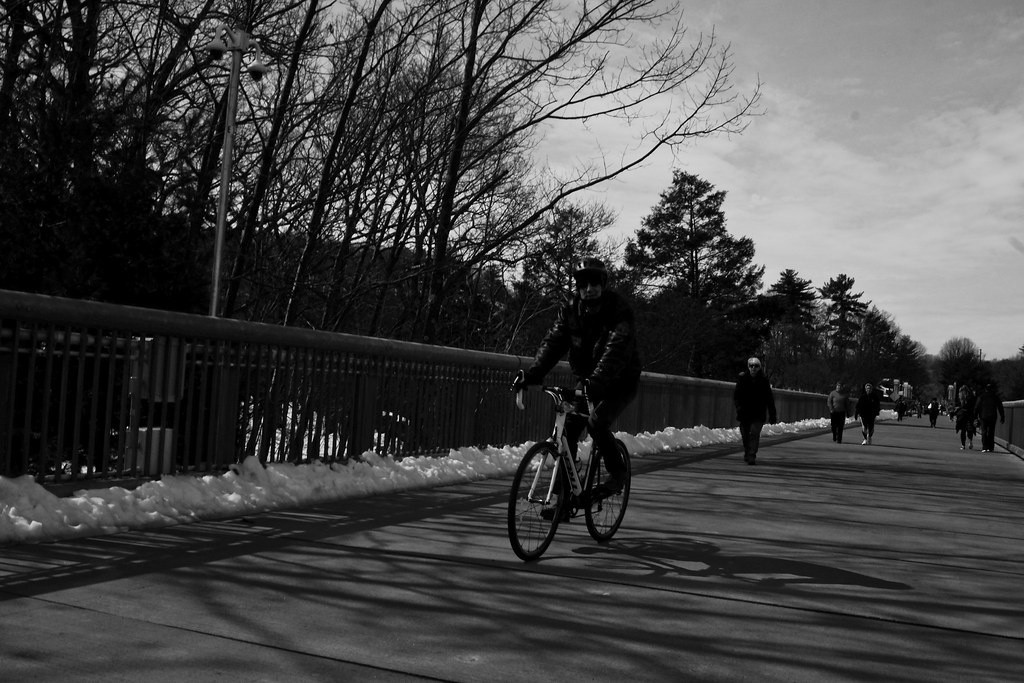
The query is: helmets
[573,259,608,289]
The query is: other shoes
[744,454,756,464]
[987,449,993,452]
[960,445,965,450]
[867,437,872,445]
[540,508,569,523]
[837,438,841,442]
[862,439,866,445]
[605,473,629,491]
[833,433,836,441]
[969,445,973,449]
[981,450,986,453]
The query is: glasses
[579,278,602,288]
[750,365,759,368]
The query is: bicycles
[507,368,632,561]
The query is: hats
[834,381,846,388]
[748,357,761,369]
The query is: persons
[513,258,641,523]
[973,384,1005,453]
[855,383,880,445]
[893,397,976,450]
[733,357,776,464]
[827,381,851,444]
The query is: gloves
[736,408,746,422]
[591,380,606,399]
[511,367,538,393]
[974,419,981,427]
[769,417,776,425]
[1000,416,1005,424]
